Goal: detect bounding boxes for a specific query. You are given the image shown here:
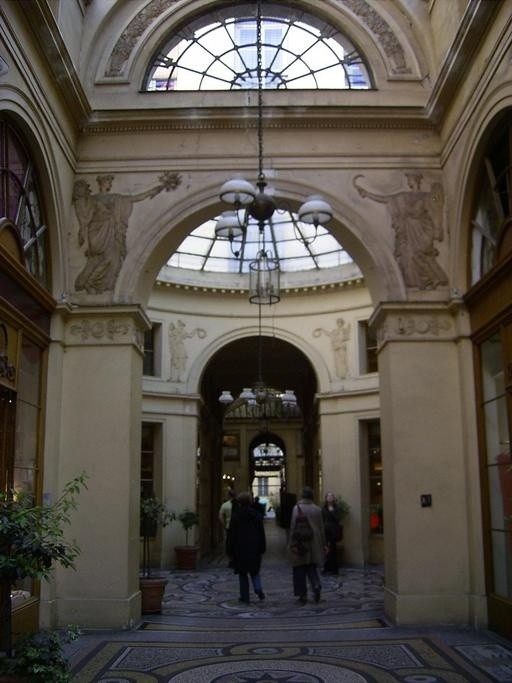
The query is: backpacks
[290,502,312,558]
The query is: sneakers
[295,595,310,605]
[237,595,249,602]
[256,588,266,602]
[312,584,322,602]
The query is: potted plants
[138,496,176,616]
[173,510,202,569]
[335,493,353,542]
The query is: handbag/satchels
[324,521,344,542]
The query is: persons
[322,491,344,575]
[217,489,237,560]
[74,172,181,295]
[287,485,329,604]
[354,169,449,291]
[225,491,267,604]
